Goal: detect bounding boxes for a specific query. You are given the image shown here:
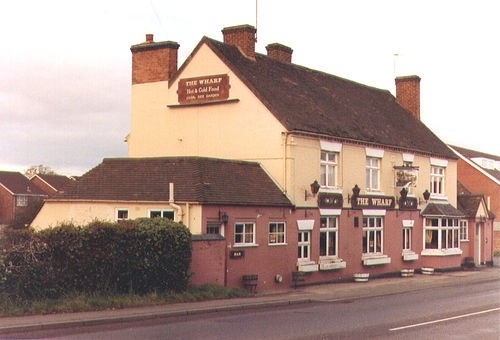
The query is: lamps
[348,184,361,204]
[418,189,430,206]
[305,180,321,201]
[218,208,229,227]
[396,187,408,204]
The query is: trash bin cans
[465,257,474,269]
[243,274,258,296]
